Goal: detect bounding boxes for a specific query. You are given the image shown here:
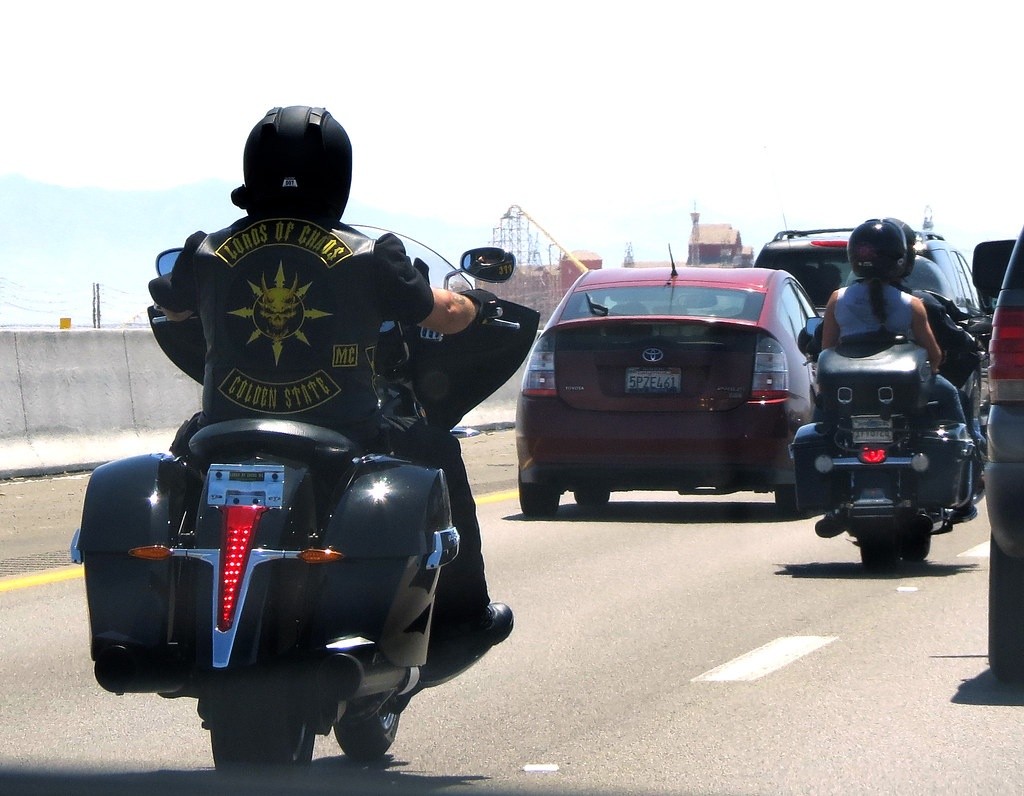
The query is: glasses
[911,248,918,257]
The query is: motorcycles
[67,223,515,776]
[787,254,993,569]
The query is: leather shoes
[429,603,513,660]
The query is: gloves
[972,337,987,356]
[458,289,501,316]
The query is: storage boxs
[915,420,976,507]
[310,453,460,666]
[71,453,203,659]
[788,421,841,516]
[816,333,933,418]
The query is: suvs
[739,222,980,369]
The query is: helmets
[848,219,908,278]
[244,105,352,224]
[884,218,916,276]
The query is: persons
[815,218,985,539]
[149,105,513,658]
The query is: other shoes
[815,515,850,538]
[956,506,979,522]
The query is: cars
[970,223,1024,683]
[515,266,823,525]
